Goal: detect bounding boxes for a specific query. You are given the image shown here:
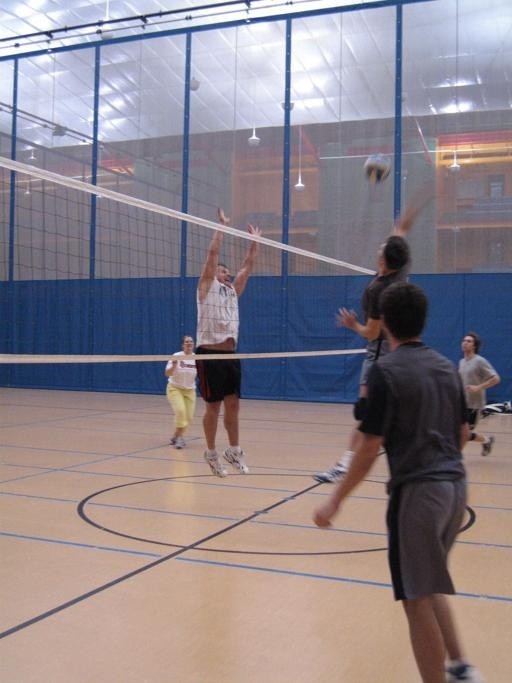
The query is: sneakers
[313,463,348,483]
[170,436,185,449]
[481,436,495,456]
[444,664,483,683]
[204,448,227,479]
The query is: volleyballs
[364,153,391,183]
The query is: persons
[312,184,430,484]
[193,207,262,478]
[313,278,486,681]
[454,330,501,456]
[164,334,198,449]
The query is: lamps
[23,176,32,198]
[190,33,199,92]
[295,20,306,191]
[449,1,460,174]
[248,75,261,147]
[26,149,36,163]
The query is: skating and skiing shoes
[222,447,250,475]
